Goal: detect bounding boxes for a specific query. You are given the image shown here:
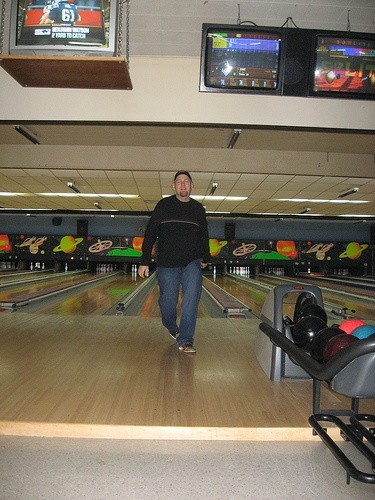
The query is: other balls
[292,315,328,353]
[311,318,375,364]
[294,303,328,324]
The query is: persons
[138,170,211,353]
[24,0,81,25]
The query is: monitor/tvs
[9,0,118,57]
[200,23,287,96]
[307,30,375,101]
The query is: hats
[175,171,191,179]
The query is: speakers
[77,220,88,238]
[224,223,235,241]
[370,226,375,245]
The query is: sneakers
[169,325,180,340]
[179,344,196,354]
